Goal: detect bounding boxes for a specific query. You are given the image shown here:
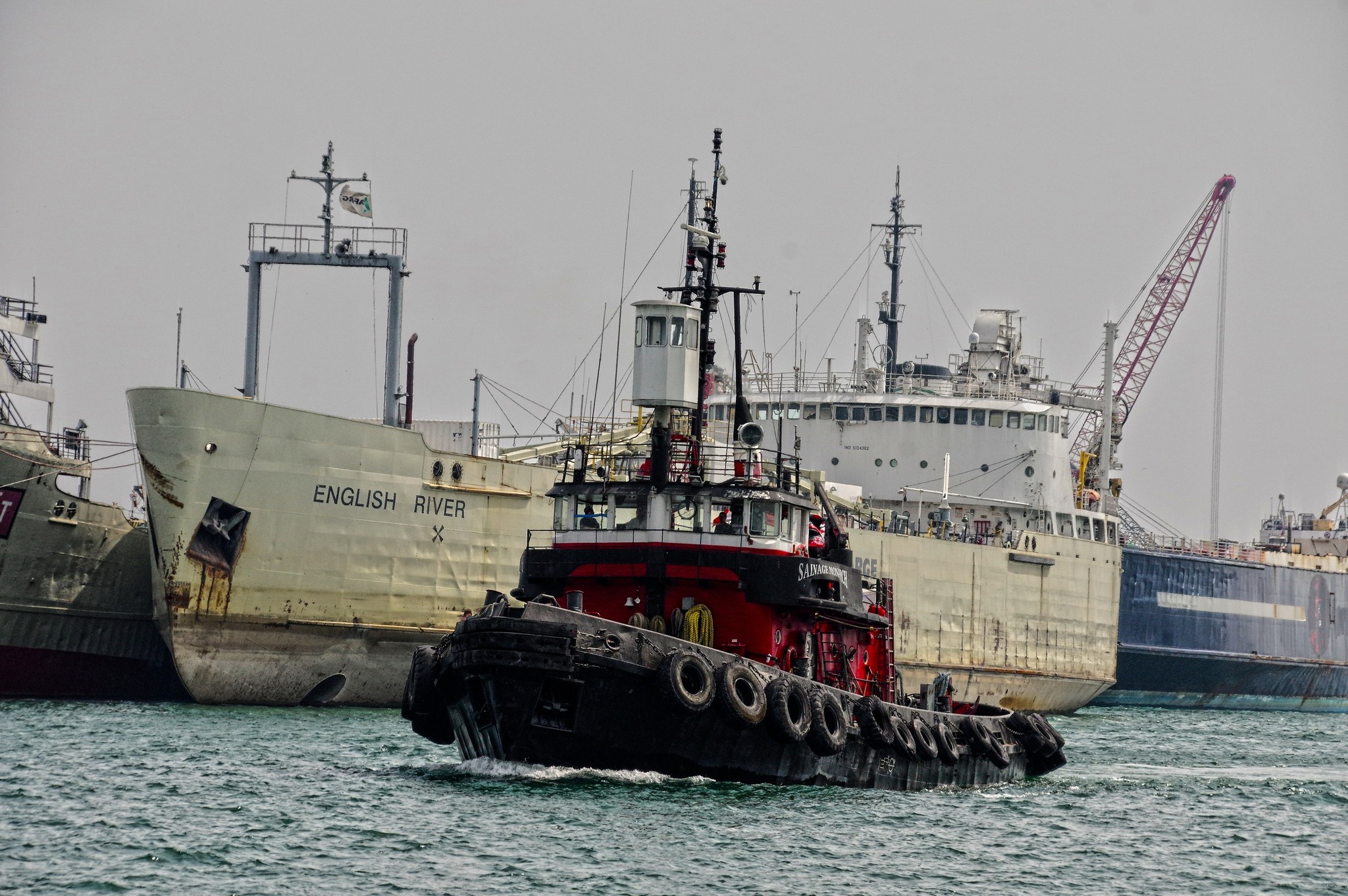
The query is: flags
[712,508,732,527]
[339,184,372,218]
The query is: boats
[126,128,1122,718]
[0,278,194,701]
[1090,473,1348,713]
[402,128,1067,789]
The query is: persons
[626,506,647,529]
[714,512,731,533]
[580,506,599,530]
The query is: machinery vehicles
[1068,174,1237,534]
